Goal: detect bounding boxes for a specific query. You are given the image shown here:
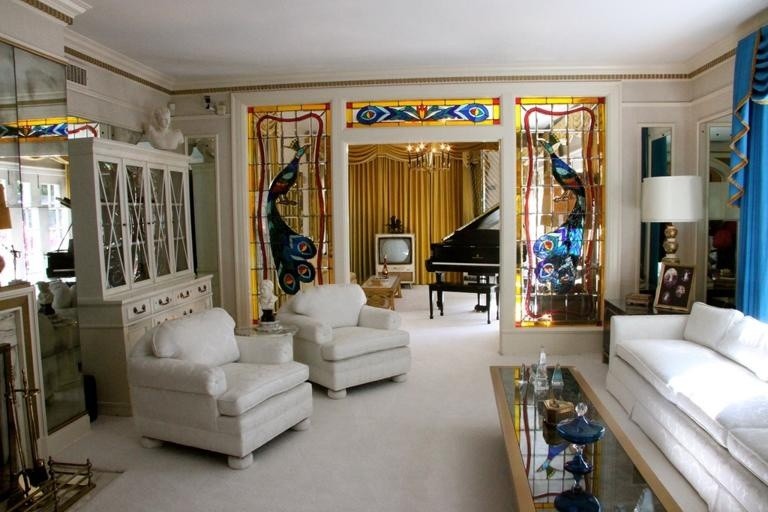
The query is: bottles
[382,257,388,278]
[515,345,565,392]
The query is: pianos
[425,205,501,323]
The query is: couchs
[605,302,768,512]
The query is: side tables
[234,324,299,337]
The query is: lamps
[407,142,452,175]
[641,175,703,266]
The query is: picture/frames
[653,262,697,313]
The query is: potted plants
[383,216,404,234]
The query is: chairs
[127,308,313,470]
[279,283,411,399]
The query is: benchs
[428,282,499,324]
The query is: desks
[604,296,692,365]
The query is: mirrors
[635,124,675,297]
[695,106,739,310]
[704,122,739,308]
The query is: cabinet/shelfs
[124,278,214,360]
[95,155,193,296]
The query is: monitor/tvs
[375,234,415,272]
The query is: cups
[167,102,176,117]
[216,104,226,115]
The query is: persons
[146,107,183,150]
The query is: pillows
[290,284,367,329]
[151,307,240,367]
[683,302,768,382]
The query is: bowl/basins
[525,294,596,321]
